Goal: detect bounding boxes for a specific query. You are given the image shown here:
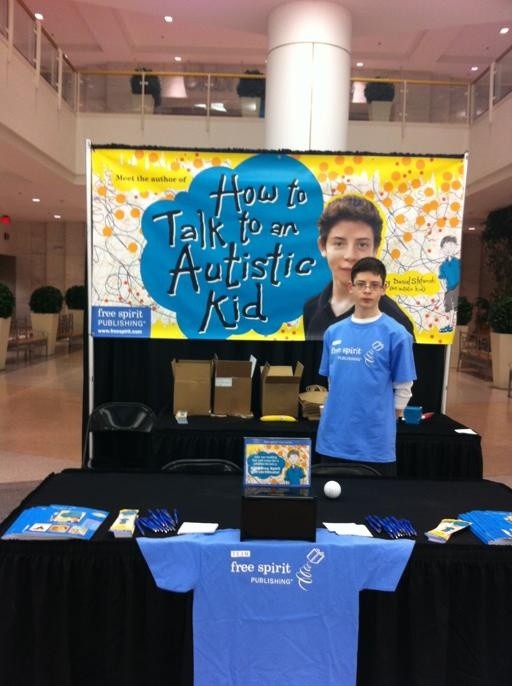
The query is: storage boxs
[215,354,258,417]
[259,352,304,423]
[172,354,213,417]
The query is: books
[2,503,110,542]
[457,510,512,546]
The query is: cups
[403,406,422,425]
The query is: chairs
[163,459,243,480]
[311,462,386,483]
[81,401,161,485]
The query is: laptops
[243,435,317,504]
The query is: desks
[88,399,482,475]
[0,468,512,684]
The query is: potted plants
[130,67,160,113]
[1,282,15,371]
[487,291,512,389]
[238,71,265,118]
[28,286,63,354]
[457,294,473,354]
[68,285,85,336]
[365,79,395,121]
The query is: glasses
[352,281,384,291]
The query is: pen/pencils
[136,507,178,535]
[365,514,418,539]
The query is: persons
[437,236,462,333]
[301,197,414,343]
[313,257,418,479]
[283,450,306,486]
